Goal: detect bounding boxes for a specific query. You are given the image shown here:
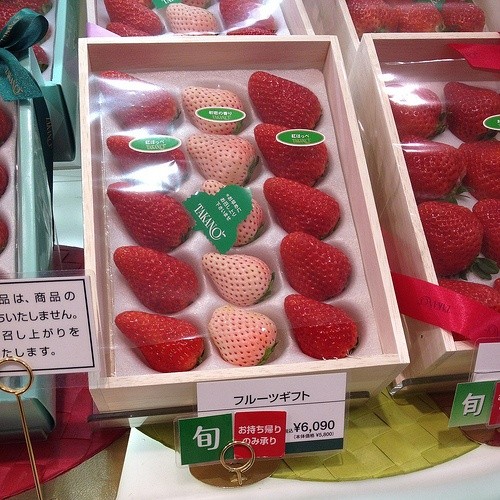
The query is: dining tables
[0,165,500,499]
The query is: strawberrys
[0,0,56,281]
[99,0,281,37]
[345,0,487,34]
[383,77,500,342]
[101,67,362,377]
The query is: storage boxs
[302,0,500,75]
[0,45,53,282]
[84,1,318,38]
[1,371,62,445]
[1,0,84,164]
[76,34,414,428]
[347,31,500,394]
